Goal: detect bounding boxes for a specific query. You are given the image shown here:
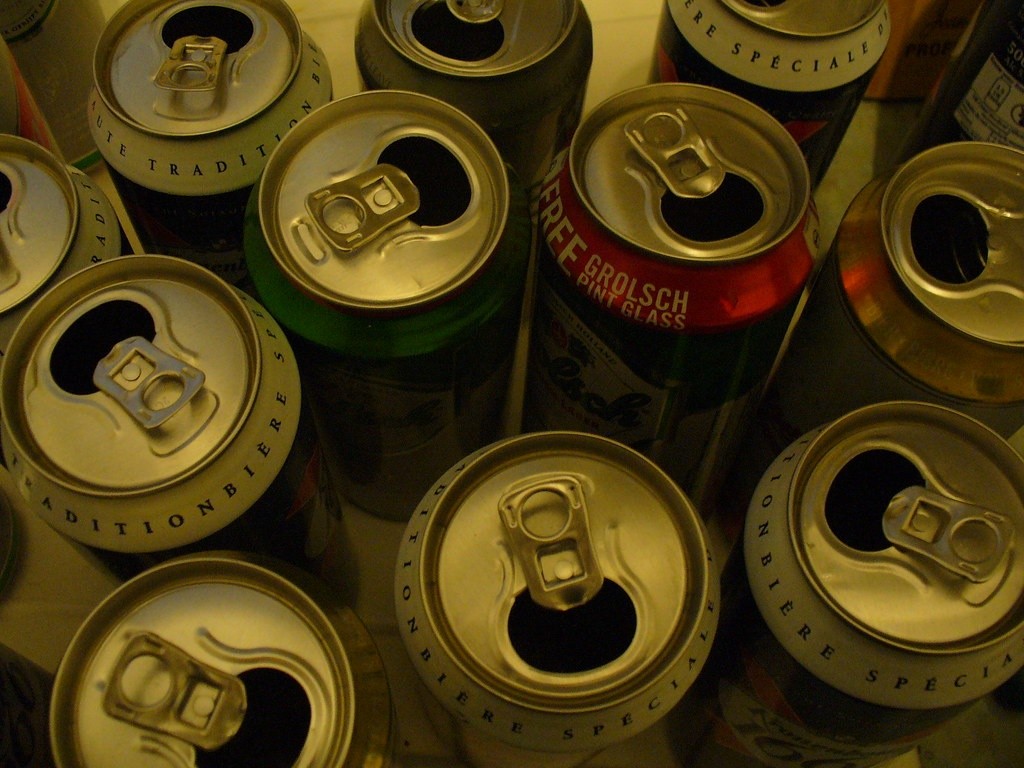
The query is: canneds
[0,0,1024,768]
